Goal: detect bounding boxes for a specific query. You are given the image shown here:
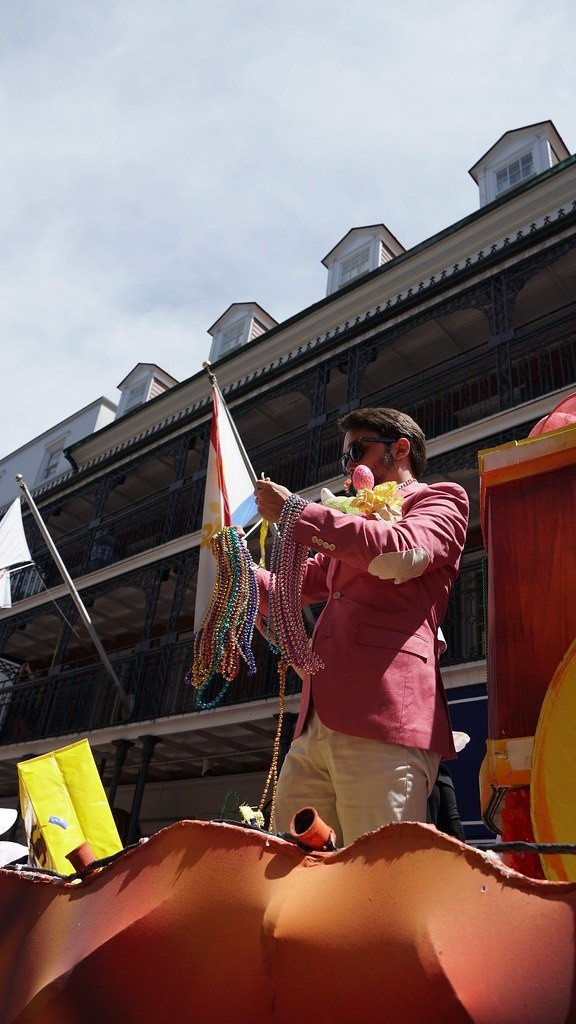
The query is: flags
[0,497,31,608]
[193,386,259,633]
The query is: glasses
[340,437,398,476]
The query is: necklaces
[258,662,285,836]
[189,526,257,708]
[398,478,416,490]
[265,493,325,676]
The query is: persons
[427,731,471,843]
[12,662,42,737]
[211,407,470,848]
[90,524,115,572]
